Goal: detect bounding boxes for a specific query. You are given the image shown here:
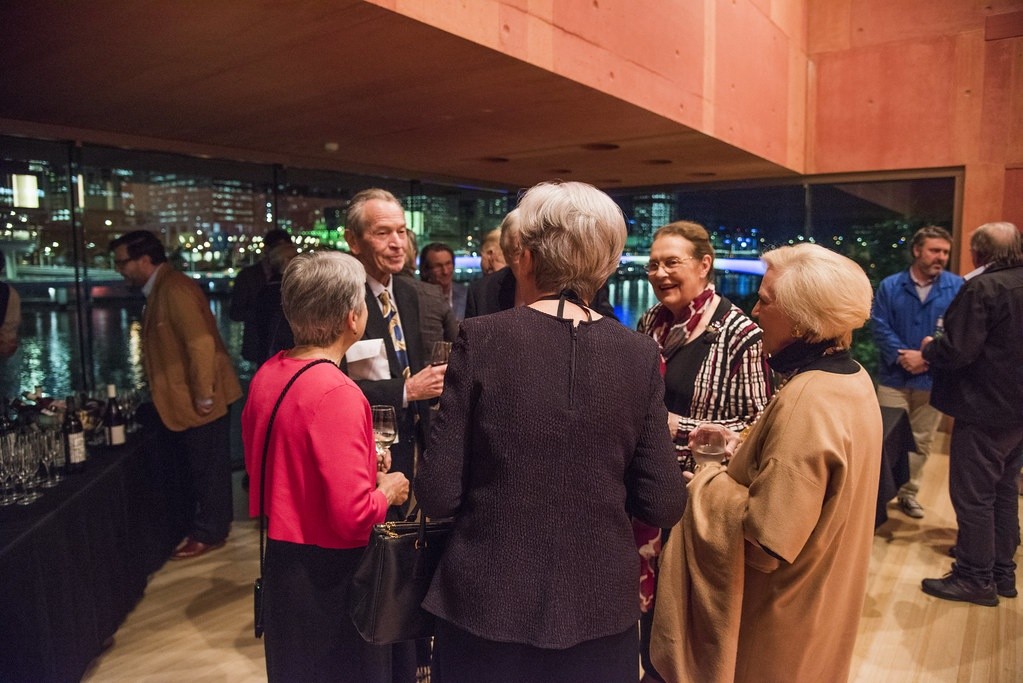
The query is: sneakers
[897,496,925,517]
[921,566,1000,607]
[951,562,1018,598]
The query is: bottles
[61,395,87,473]
[934,315,945,337]
[102,381,126,447]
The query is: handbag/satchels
[347,504,454,645]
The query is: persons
[107,230,245,560]
[919,222,1023,608]
[226,226,306,370]
[401,230,463,442]
[237,253,409,683]
[318,188,451,683]
[866,223,965,518]
[425,180,882,683]
[0,246,24,368]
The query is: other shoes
[949,546,957,556]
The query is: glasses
[644,252,706,276]
[506,249,522,264]
[113,257,131,269]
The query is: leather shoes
[169,536,227,560]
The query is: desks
[0,401,192,682]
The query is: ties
[377,290,420,425]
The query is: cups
[691,422,727,466]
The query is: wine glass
[427,341,452,411]
[0,415,66,507]
[87,388,145,447]
[370,405,398,471]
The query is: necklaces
[735,346,834,458]
